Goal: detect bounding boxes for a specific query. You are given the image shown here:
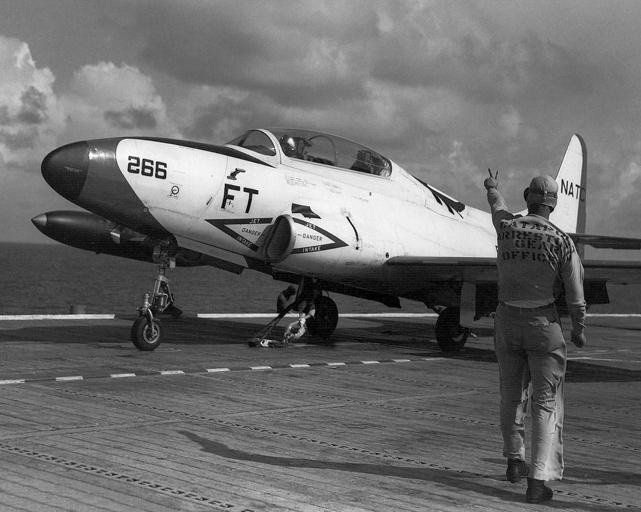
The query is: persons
[484,168,587,503]
[279,135,298,158]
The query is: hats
[523,175,558,213]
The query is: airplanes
[31,129,641,352]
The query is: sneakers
[506,459,530,484]
[525,479,553,504]
